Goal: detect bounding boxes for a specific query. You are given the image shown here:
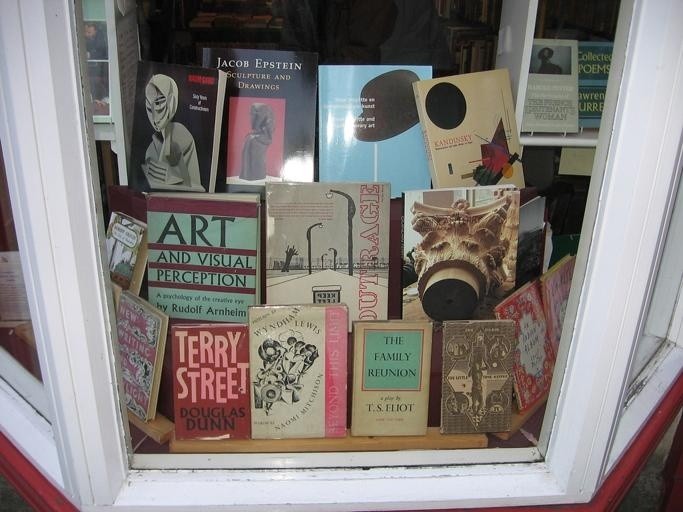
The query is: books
[82,3,613,328]
[118,291,170,426]
[352,321,433,435]
[491,254,576,415]
[0,251,33,331]
[441,320,511,432]
[170,324,250,441]
[248,305,347,438]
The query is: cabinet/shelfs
[76,0,139,186]
[493,0,632,147]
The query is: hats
[352,70,420,141]
